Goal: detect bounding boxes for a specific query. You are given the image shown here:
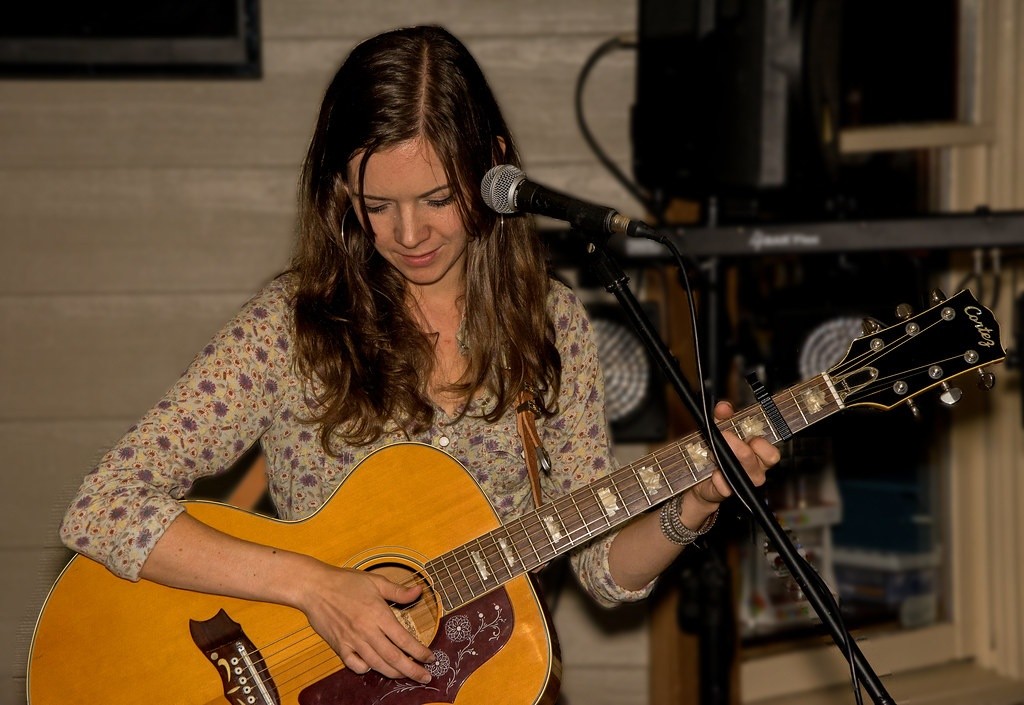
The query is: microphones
[480,164,666,244]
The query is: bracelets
[658,492,720,547]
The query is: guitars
[25,289,1004,705]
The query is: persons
[60,22,786,705]
